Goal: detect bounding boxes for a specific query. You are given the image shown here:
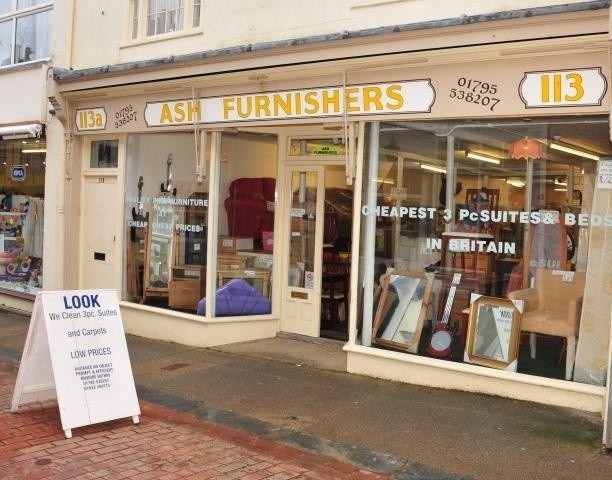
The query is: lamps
[509,127,545,161]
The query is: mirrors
[139,191,178,307]
[463,291,524,374]
[372,266,436,355]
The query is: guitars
[427,272,462,360]
[161,151,176,198]
[130,175,149,242]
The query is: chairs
[322,273,349,332]
[440,188,501,277]
[508,266,587,381]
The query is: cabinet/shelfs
[168,265,202,310]
[0,212,26,275]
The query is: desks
[435,272,508,353]
[217,268,270,298]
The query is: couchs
[224,177,277,242]
